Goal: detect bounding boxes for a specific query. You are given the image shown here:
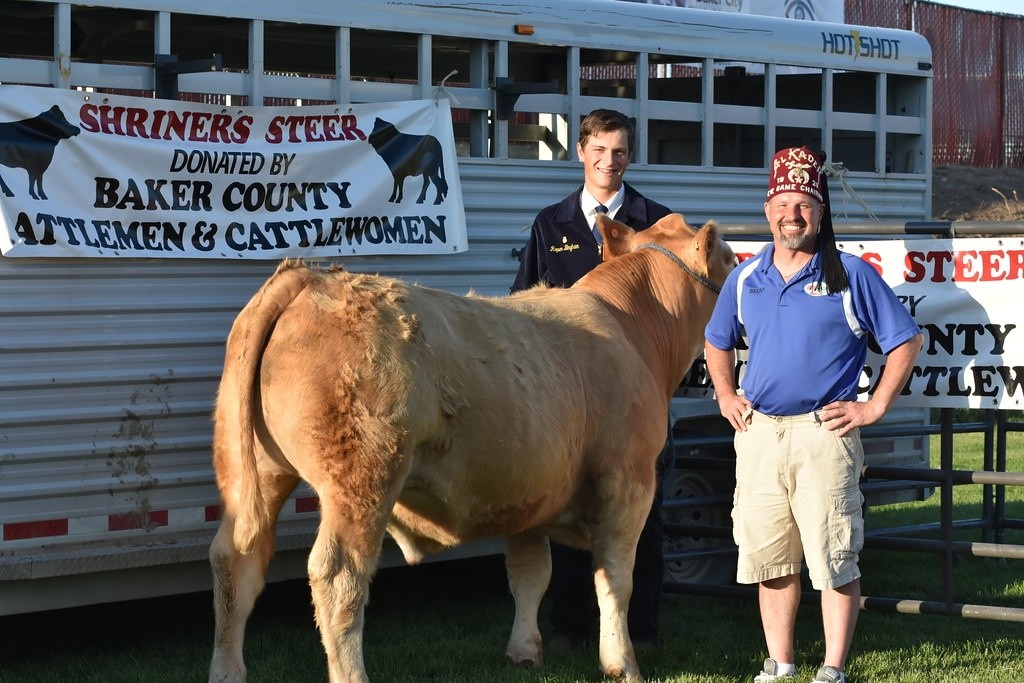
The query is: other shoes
[553,604,590,639]
[629,604,661,642]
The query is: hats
[767,147,824,205]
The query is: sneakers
[753,658,794,682]
[813,664,844,683]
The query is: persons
[701,149,925,681]
[511,110,688,633]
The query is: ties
[594,206,609,249]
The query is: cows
[207,211,741,683]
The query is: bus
[0,0,946,650]
